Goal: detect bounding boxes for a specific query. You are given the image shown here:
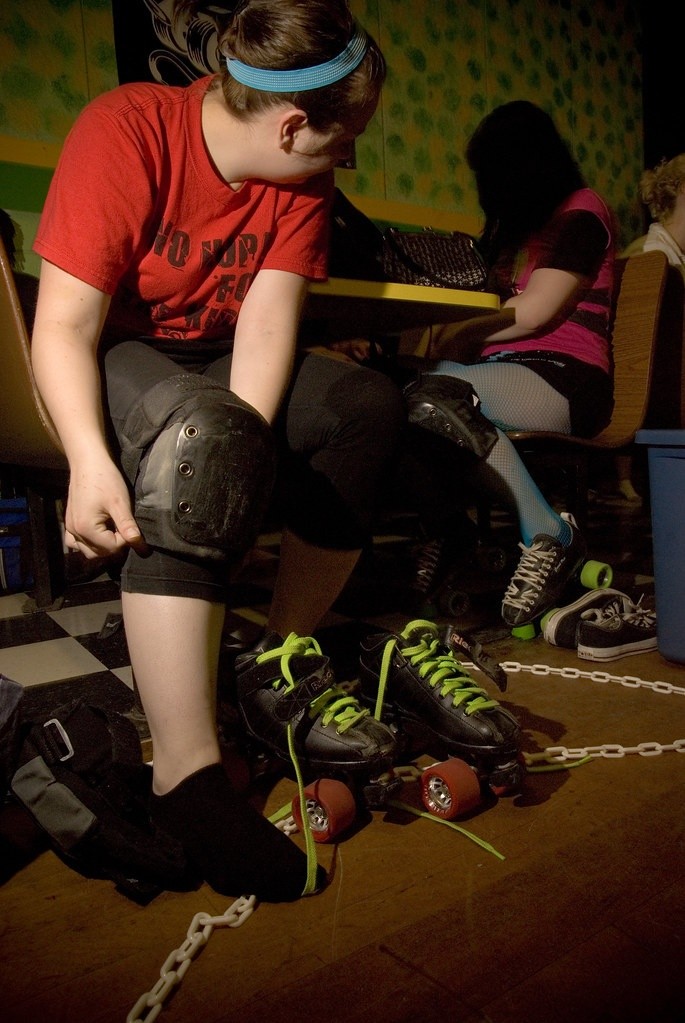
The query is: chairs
[476,250,669,540]
[0,231,69,612]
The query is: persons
[614,151,685,283]
[404,99,613,639]
[30,0,416,901]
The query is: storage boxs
[634,430,685,664]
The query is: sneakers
[577,609,658,663]
[543,586,635,647]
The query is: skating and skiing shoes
[354,619,527,821]
[500,512,613,634]
[402,513,510,618]
[219,631,398,845]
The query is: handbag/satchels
[375,226,489,291]
[7,706,199,895]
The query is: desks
[289,276,501,349]
